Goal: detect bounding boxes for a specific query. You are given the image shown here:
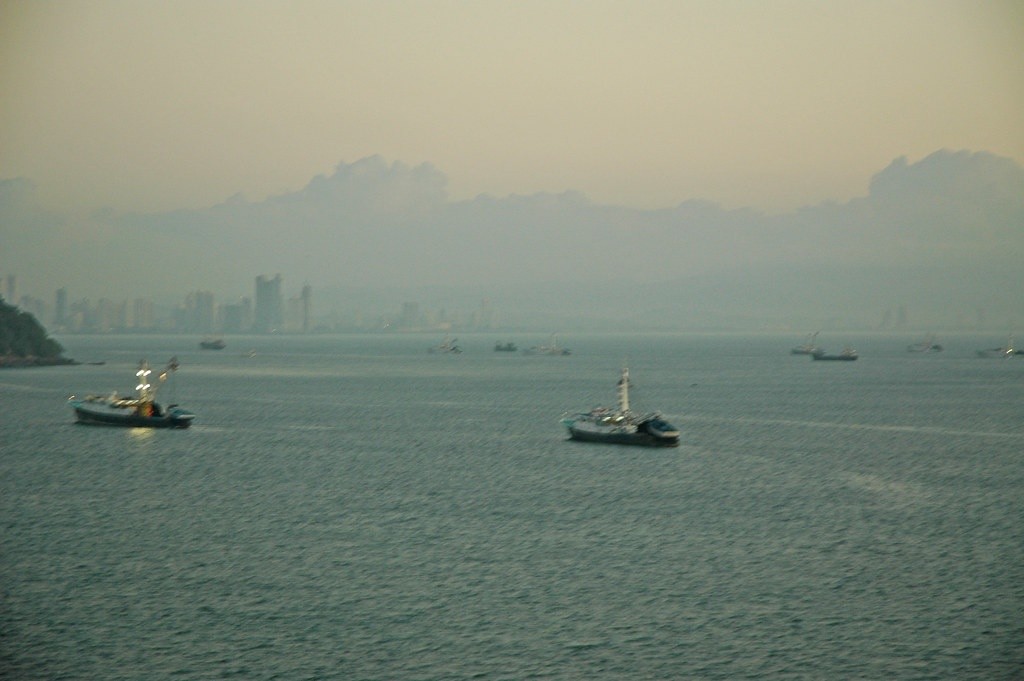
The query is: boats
[71,359,198,425]
[562,372,683,446]
